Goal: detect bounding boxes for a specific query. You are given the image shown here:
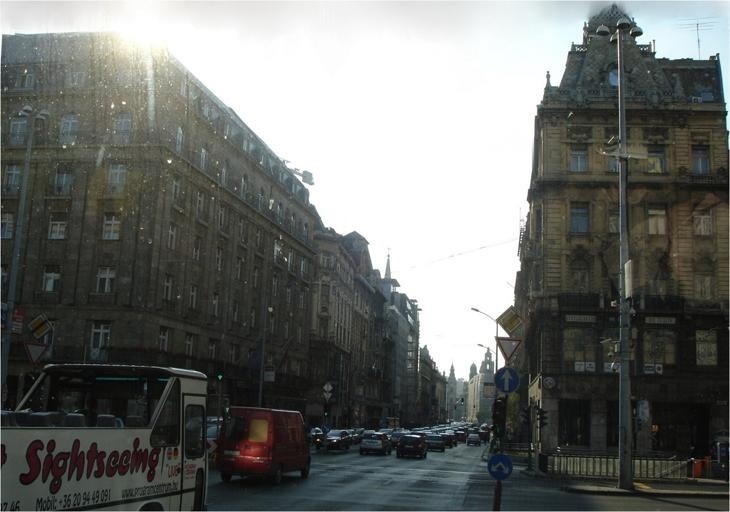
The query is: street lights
[1,105,51,403]
[477,343,497,355]
[471,306,498,404]
[598,15,646,490]
[257,303,275,407]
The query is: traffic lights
[324,405,328,417]
[520,406,532,425]
[492,398,506,438]
[461,398,465,406]
[215,369,224,383]
[537,408,549,429]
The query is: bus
[2,361,209,511]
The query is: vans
[217,407,311,485]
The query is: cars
[310,420,493,459]
[184,415,223,452]
